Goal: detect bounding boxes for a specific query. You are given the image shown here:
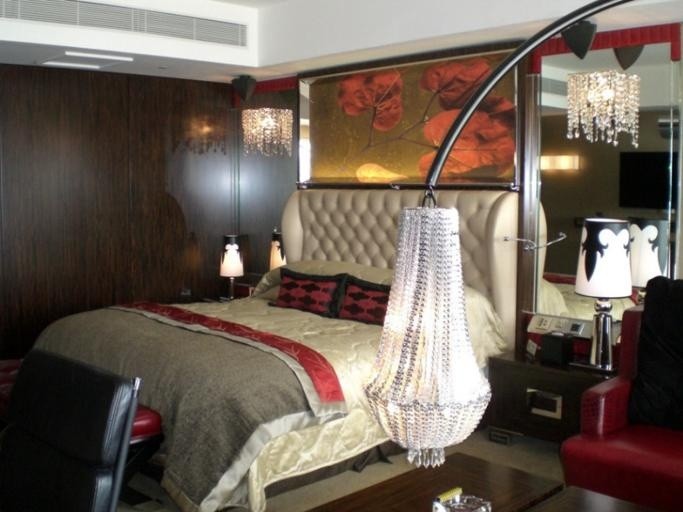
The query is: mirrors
[515,22,680,368]
[239,77,300,286]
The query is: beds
[36,183,523,512]
[540,204,569,317]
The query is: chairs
[1,348,142,512]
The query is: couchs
[126,404,165,482]
[561,307,683,512]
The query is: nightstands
[486,315,620,443]
[197,278,255,303]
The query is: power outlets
[536,316,552,328]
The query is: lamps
[568,70,639,150]
[574,217,631,369]
[363,1,682,468]
[242,110,292,158]
[221,233,245,298]
[171,103,228,156]
[629,215,671,304]
[269,234,287,271]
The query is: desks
[307,452,673,512]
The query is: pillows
[268,268,345,318]
[339,275,391,326]
